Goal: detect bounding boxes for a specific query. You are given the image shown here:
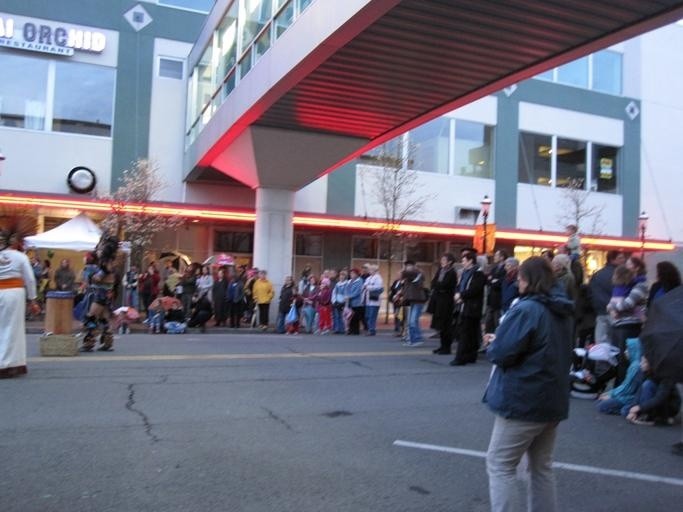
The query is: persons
[124,264,275,335]
[79,232,119,351]
[53,257,74,293]
[30,255,42,292]
[0,231,40,381]
[41,258,53,301]
[480,254,576,511]
[276,264,383,336]
[393,226,682,423]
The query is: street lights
[479,194,493,255]
[637,210,651,261]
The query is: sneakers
[217,323,377,335]
[402,340,480,365]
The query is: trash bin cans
[45,291,77,334]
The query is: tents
[23,209,132,272]
